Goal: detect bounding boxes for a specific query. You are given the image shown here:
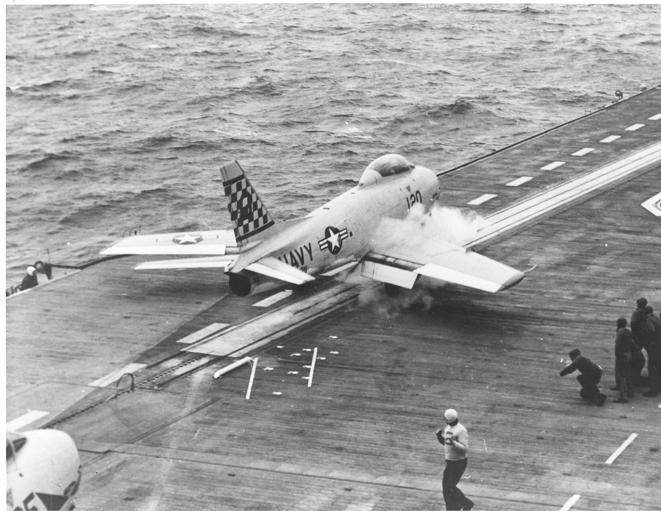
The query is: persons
[432,408,474,511]
[558,297,660,407]
[18,265,38,289]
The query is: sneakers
[609,386,659,402]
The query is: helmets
[443,408,458,424]
